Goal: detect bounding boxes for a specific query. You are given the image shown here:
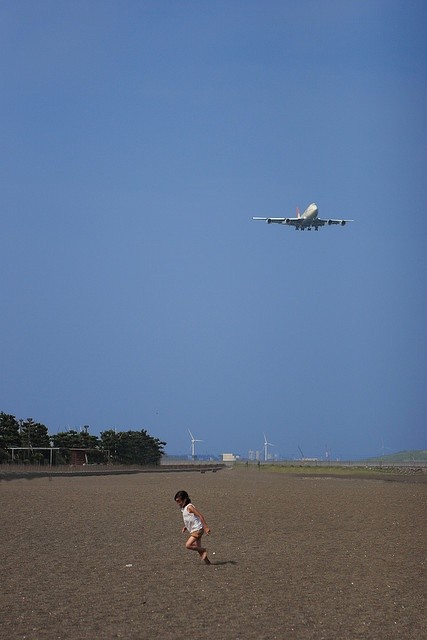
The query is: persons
[173,490,212,567]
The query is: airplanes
[251,202,354,231]
